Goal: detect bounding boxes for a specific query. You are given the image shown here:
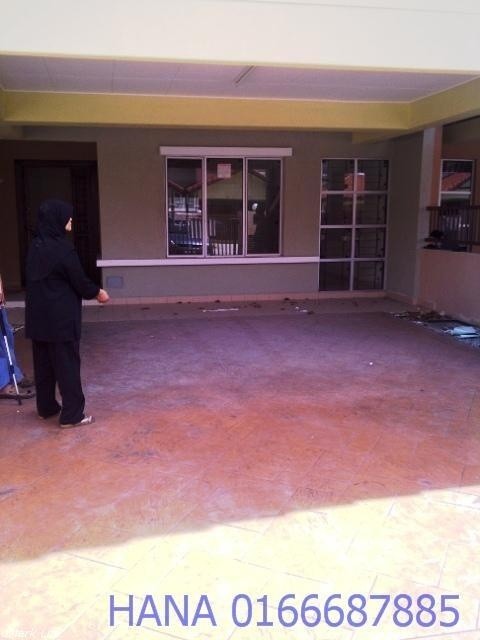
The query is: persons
[23,199,110,428]
[0,275,35,399]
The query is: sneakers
[19,377,35,388]
[60,415,92,428]
[0,384,35,399]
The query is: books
[168,195,239,256]
